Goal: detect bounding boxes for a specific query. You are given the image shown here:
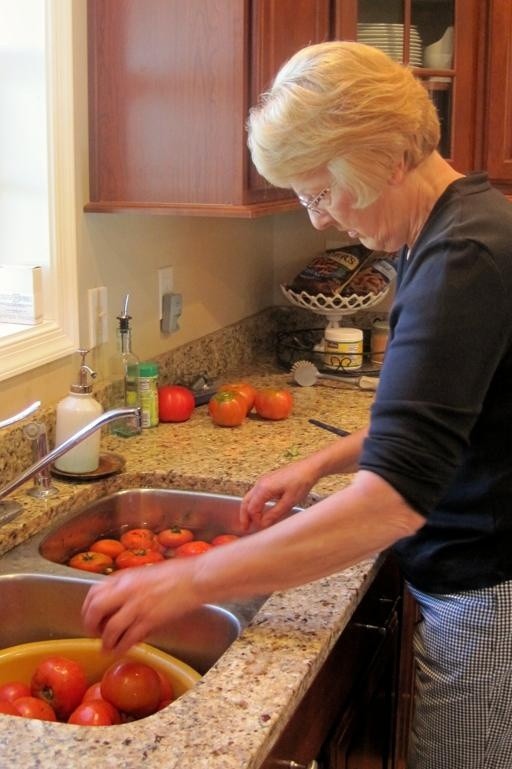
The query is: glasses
[296,183,332,215]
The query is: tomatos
[158,385,195,423]
[208,383,293,427]
[69,527,239,574]
[0,657,174,727]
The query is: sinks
[37,488,316,579]
[1,575,241,727]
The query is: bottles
[124,361,158,429]
[108,312,144,435]
[370,320,391,364]
[325,326,363,370]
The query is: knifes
[307,417,350,438]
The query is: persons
[81,37,511,769]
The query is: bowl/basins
[1,637,203,697]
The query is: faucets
[0,407,143,526]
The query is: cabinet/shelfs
[474,2,512,193]
[250,550,397,768]
[85,0,481,223]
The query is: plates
[356,22,425,81]
[50,450,127,479]
